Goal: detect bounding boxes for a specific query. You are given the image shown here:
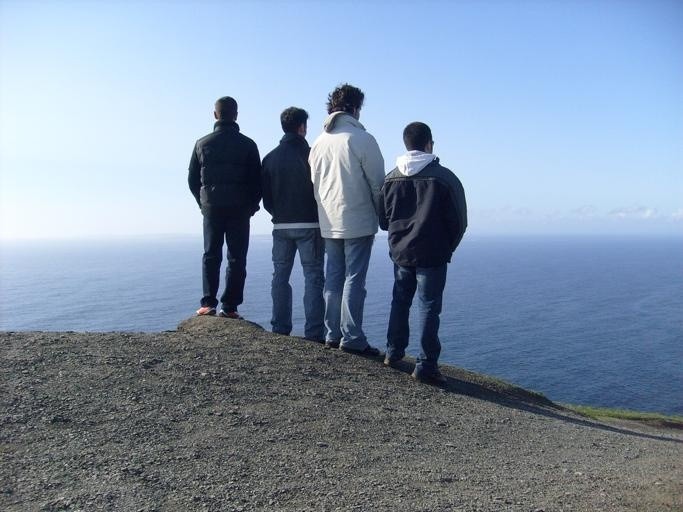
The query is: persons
[304,81,387,356]
[260,105,326,342]
[369,121,468,385]
[186,95,263,319]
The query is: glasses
[428,140,435,145]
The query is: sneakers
[412,365,449,385]
[195,307,218,316]
[325,341,340,347]
[337,343,380,357]
[217,308,246,320]
[384,355,404,365]
